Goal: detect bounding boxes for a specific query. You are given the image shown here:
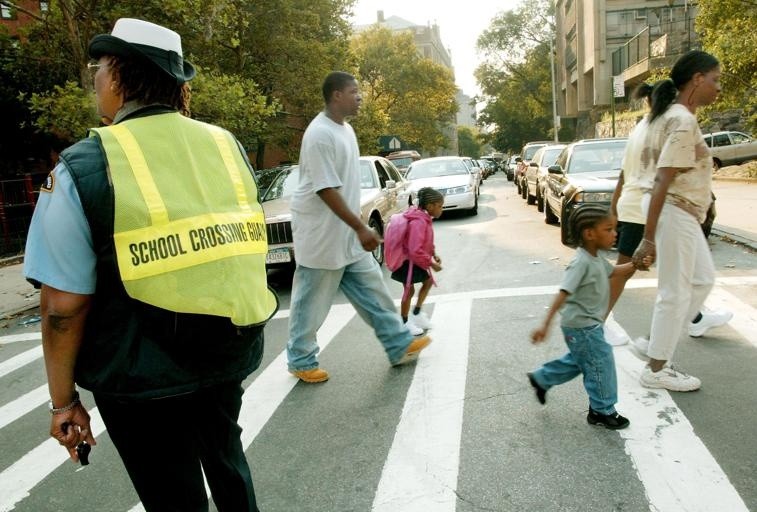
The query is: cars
[703,130,755,168]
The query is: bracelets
[641,238,655,247]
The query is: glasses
[87,16,196,84]
[87,61,110,74]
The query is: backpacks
[384,212,427,273]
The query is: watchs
[48,391,80,416]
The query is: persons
[526,202,653,430]
[383,186,444,337]
[284,72,431,383]
[21,19,281,512]
[630,50,735,394]
[602,77,734,346]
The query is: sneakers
[403,320,424,336]
[686,310,733,337]
[411,313,434,329]
[637,365,702,393]
[287,367,329,383]
[629,337,652,362]
[586,406,628,430]
[525,370,546,405]
[602,324,629,348]
[391,336,431,367]
